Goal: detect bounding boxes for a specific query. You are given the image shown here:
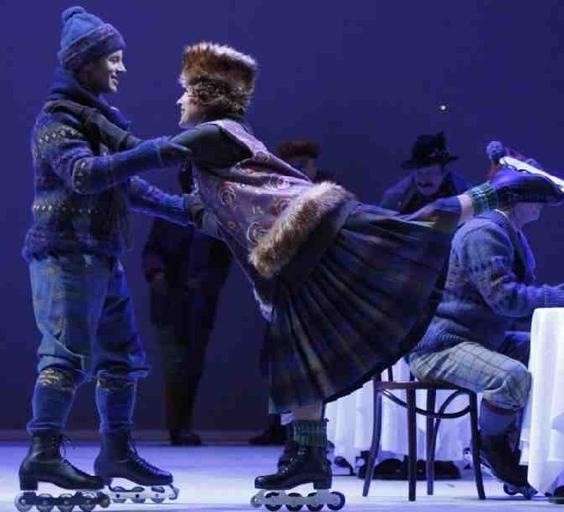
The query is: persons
[377,129,478,215]
[42,38,563,490]
[249,138,359,446]
[406,139,564,499]
[139,163,236,446]
[17,5,196,491]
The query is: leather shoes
[172,434,199,446]
[250,429,281,444]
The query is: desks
[526,307,562,505]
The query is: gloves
[43,99,92,125]
[160,136,193,159]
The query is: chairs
[362,353,488,503]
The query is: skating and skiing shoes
[15,431,177,511]
[491,157,564,207]
[250,446,345,511]
[464,431,536,499]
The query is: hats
[486,141,541,176]
[178,42,256,114]
[58,6,125,69]
[400,131,458,169]
[278,140,319,158]
